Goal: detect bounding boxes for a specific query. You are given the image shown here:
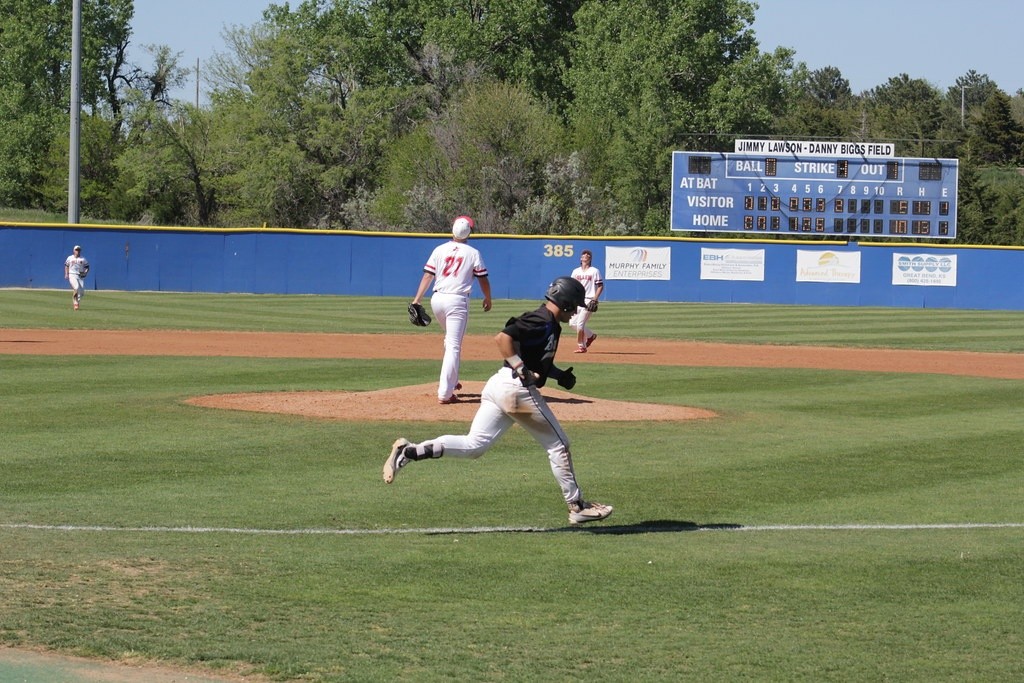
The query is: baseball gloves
[586,300,598,312]
[407,303,432,327]
[78,272,87,279]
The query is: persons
[64,245,89,311]
[570,249,605,353]
[407,215,492,405]
[382,276,614,525]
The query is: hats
[452,218,471,240]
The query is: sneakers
[383,438,408,484]
[569,500,613,524]
[586,334,597,348]
[454,383,462,390]
[438,394,458,404]
[574,346,587,353]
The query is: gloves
[517,367,540,387]
[557,367,576,390]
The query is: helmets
[546,276,588,313]
